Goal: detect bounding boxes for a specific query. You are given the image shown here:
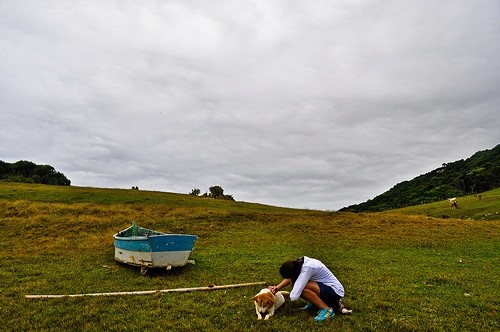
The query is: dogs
[252,288,290,320]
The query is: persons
[267,256,344,321]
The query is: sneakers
[314,308,334,321]
[299,304,312,310]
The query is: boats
[113,220,198,274]
